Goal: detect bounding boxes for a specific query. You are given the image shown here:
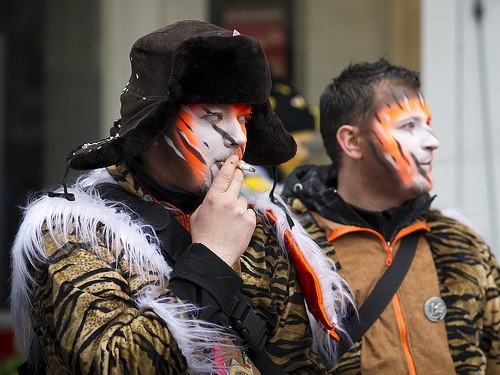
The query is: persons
[267,78,319,177]
[282,60,500,375]
[10,20,358,375]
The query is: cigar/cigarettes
[237,162,257,172]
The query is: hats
[46,20,298,202]
